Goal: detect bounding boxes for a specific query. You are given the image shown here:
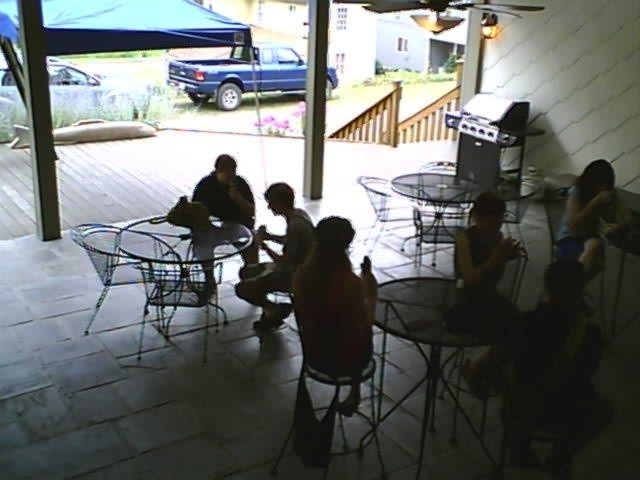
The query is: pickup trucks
[167,38,342,110]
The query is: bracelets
[260,242,267,250]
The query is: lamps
[481,14,502,39]
[409,12,465,34]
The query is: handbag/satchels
[149,196,210,229]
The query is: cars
[0,51,155,131]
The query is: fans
[332,0,545,19]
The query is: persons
[290,215,380,418]
[552,158,619,302]
[190,154,260,294]
[507,260,614,479]
[233,181,314,329]
[451,191,529,402]
